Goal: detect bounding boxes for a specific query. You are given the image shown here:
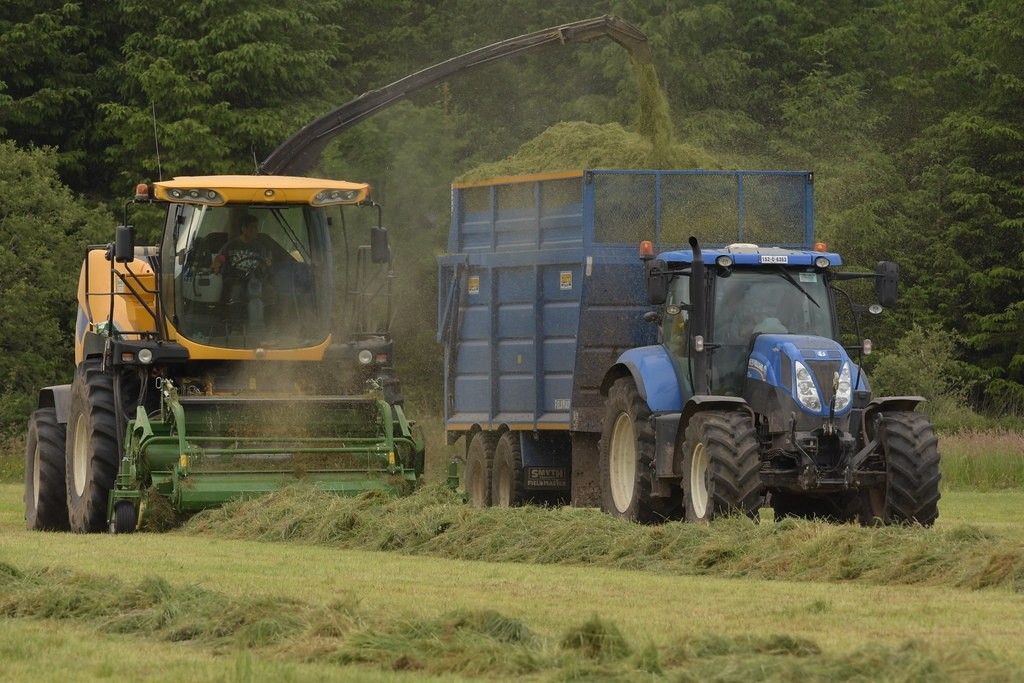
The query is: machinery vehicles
[22,13,649,542]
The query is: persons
[210,215,279,336]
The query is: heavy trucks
[431,167,944,529]
